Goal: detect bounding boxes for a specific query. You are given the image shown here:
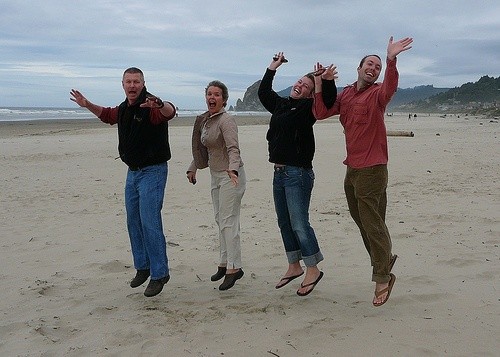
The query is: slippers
[297,271,324,296]
[388,254,397,272]
[372,273,396,307]
[275,271,304,290]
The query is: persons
[186,80,246,291]
[314,36,413,307]
[257,52,338,296]
[69,67,177,297]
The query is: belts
[274,165,299,172]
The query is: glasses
[273,56,288,63]
[313,69,326,76]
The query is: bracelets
[155,102,164,109]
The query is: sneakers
[130,269,150,288]
[219,268,244,291]
[144,275,169,297]
[211,265,227,282]
